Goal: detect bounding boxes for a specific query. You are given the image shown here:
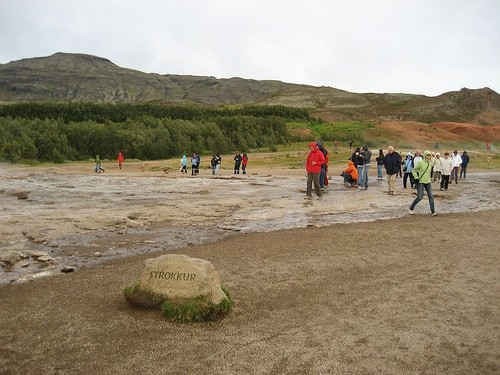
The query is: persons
[234,152,241,174]
[400,150,469,191]
[304,143,325,199]
[117,152,124,170]
[190,152,200,175]
[342,162,359,186]
[349,146,370,188]
[95,155,106,173]
[242,153,248,174]
[211,154,221,174]
[383,145,402,194]
[180,152,187,173]
[376,149,385,180]
[316,143,328,191]
[409,151,438,217]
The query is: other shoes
[317,194,323,200]
[409,209,415,214]
[432,212,438,216]
[304,195,312,200]
[321,188,324,191]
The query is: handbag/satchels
[412,172,419,184]
[324,176,328,186]
[403,167,407,171]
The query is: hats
[453,151,458,153]
[444,153,449,155]
